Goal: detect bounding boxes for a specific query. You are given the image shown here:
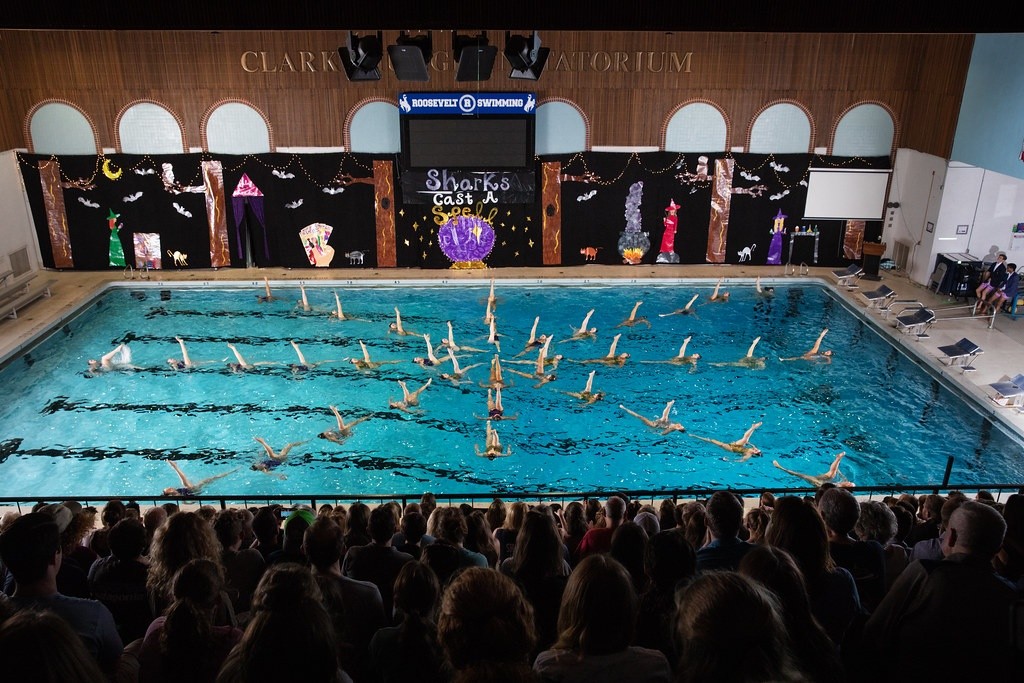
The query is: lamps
[387,30,433,81]
[452,30,498,82]
[502,31,550,82]
[337,30,384,82]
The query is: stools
[1016,407,1024,416]
[915,333,930,342]
[878,308,892,316]
[847,284,860,292]
[960,367,976,376]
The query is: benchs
[0,278,58,321]
[0,270,15,286]
[0,273,37,302]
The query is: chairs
[895,307,935,335]
[988,373,1024,404]
[860,285,894,309]
[831,264,863,286]
[936,338,980,367]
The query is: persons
[1,491,1024,682]
[477,297,499,326]
[160,458,245,506]
[474,313,514,352]
[504,275,861,498]
[976,255,1008,314]
[475,421,513,462]
[978,263,1023,326]
[318,405,375,445]
[483,278,501,311]
[474,383,520,422]
[389,377,434,415]
[250,435,303,480]
[479,355,515,391]
[435,320,490,354]
[88,275,475,379]
[440,347,489,385]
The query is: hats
[36,501,82,534]
[284,510,315,543]
[633,512,661,538]
